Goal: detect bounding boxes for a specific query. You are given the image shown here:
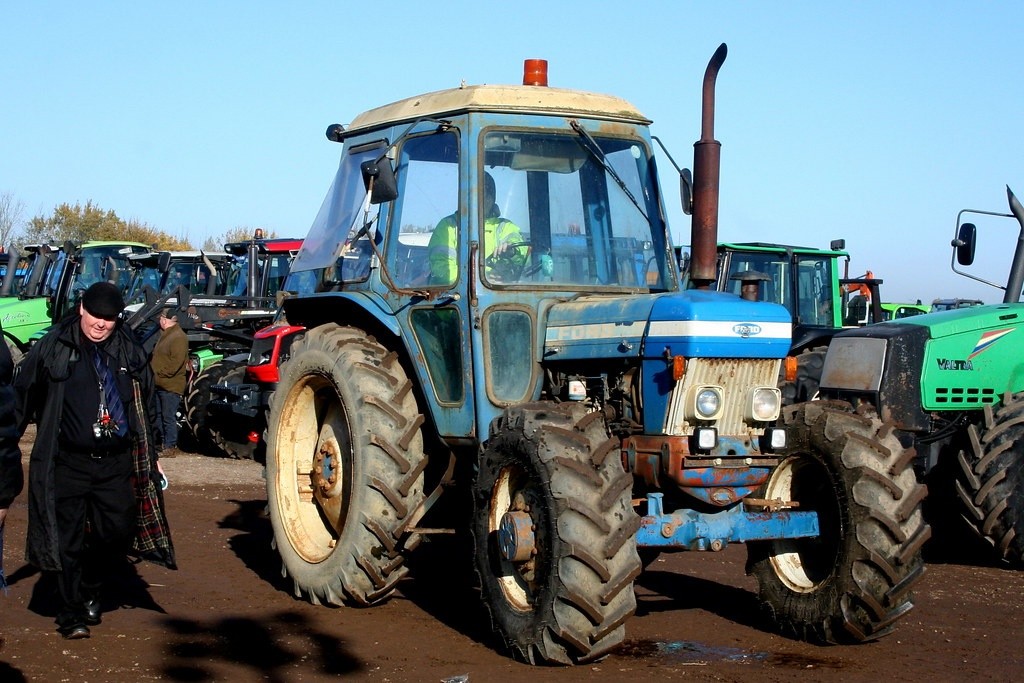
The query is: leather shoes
[73,574,102,624]
[56,606,90,639]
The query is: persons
[428,171,531,322]
[12,282,177,638]
[150,308,188,459]
[0,323,24,589]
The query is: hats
[484,171,496,203]
[186,306,197,315]
[156,308,178,322]
[82,282,126,317]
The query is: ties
[91,342,128,438]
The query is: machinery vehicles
[261,41,932,667]
[0,235,984,468]
[805,182,1024,568]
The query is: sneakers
[157,446,177,459]
